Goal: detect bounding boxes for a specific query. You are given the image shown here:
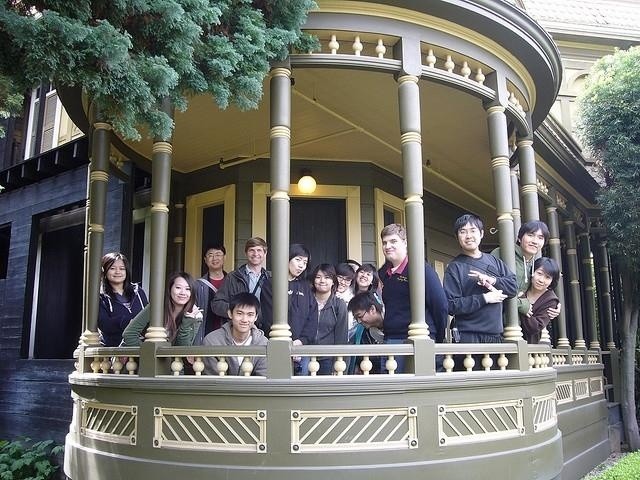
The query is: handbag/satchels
[111,332,144,366]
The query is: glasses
[337,275,352,284]
[354,305,371,320]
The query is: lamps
[297,167,317,195]
